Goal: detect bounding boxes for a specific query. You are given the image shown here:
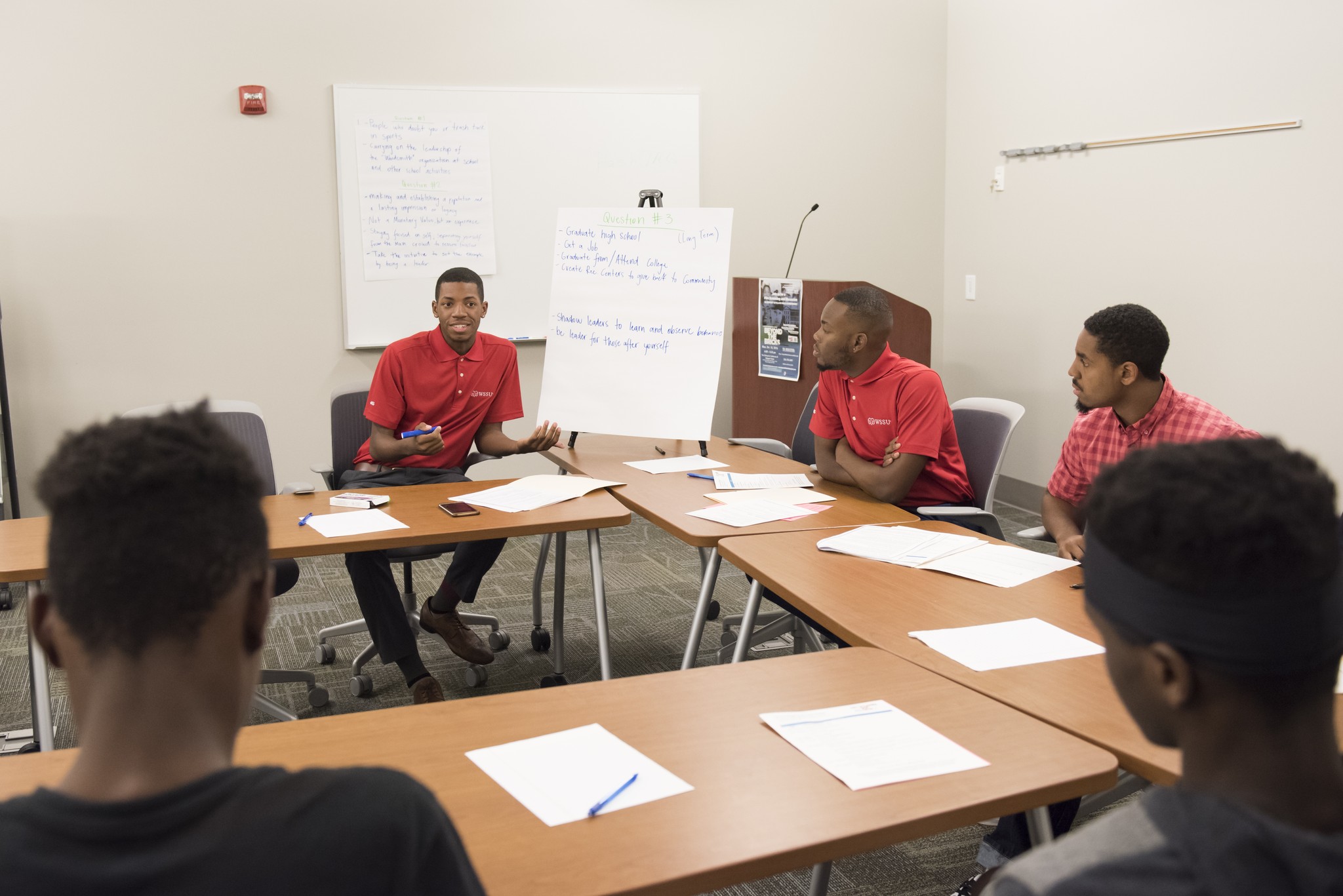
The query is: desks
[1,478,635,756]
[1,643,1118,895]
[715,521,1343,792]
[531,429,921,680]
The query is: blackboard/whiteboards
[333,82,701,350]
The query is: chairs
[318,386,510,700]
[700,380,831,669]
[899,397,1027,544]
[212,406,332,730]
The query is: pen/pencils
[298,513,313,526]
[687,473,714,480]
[588,773,639,818]
[655,446,665,455]
[1070,582,1085,589]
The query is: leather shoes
[419,596,495,665]
[951,866,1002,896]
[410,676,445,705]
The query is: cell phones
[439,501,480,517]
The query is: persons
[947,302,1261,896]
[761,284,799,342]
[336,266,565,705]
[975,437,1343,896]
[809,289,976,533]
[1,408,489,896]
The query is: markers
[543,336,547,338]
[508,337,530,341]
[401,426,439,439]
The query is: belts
[354,462,404,474]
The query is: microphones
[784,203,819,278]
